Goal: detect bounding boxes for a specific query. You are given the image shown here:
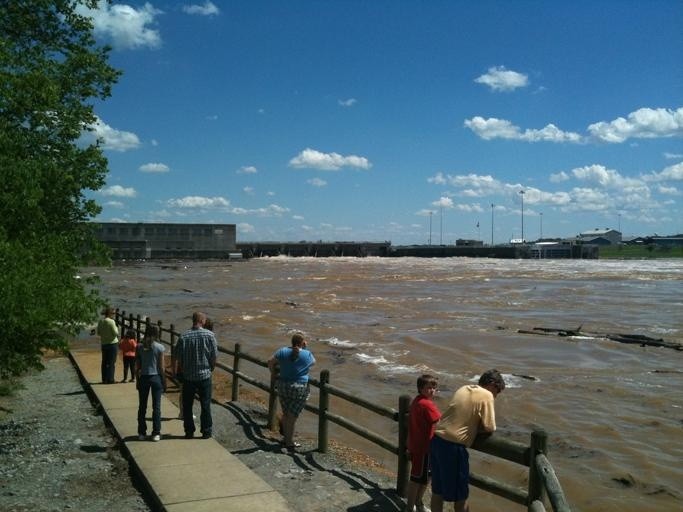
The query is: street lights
[617,214,623,233]
[539,212,544,238]
[518,190,525,244]
[491,203,494,245]
[428,211,433,245]
[475,221,480,240]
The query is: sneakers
[102,379,135,384]
[281,442,300,447]
[138,434,160,442]
[185,432,211,439]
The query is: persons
[180,317,213,420]
[97,307,119,383]
[172,311,217,439]
[406,374,443,512]
[120,329,138,383]
[135,325,167,442]
[267,332,316,448]
[430,370,506,512]
